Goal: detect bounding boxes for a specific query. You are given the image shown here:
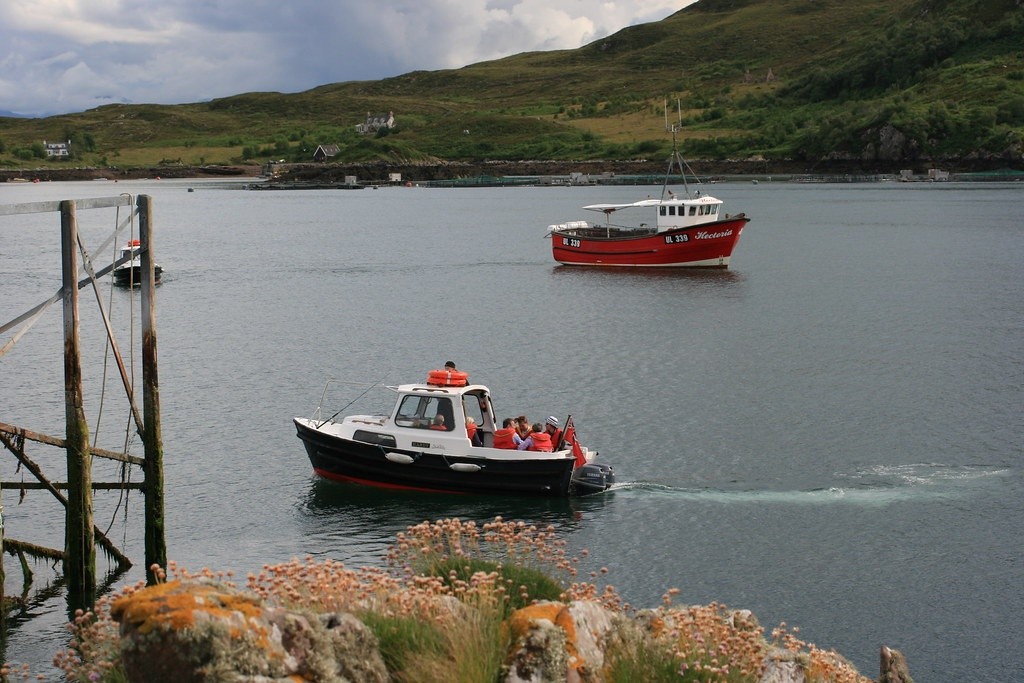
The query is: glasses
[546,423,549,425]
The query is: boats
[111,237,164,286]
[293,365,615,500]
[545,97,752,270]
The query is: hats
[445,361,455,368]
[532,422,543,431]
[546,416,558,427]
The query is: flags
[562,417,587,469]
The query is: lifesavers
[128,240,140,247]
[427,378,466,386]
[428,370,467,379]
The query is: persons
[493,418,523,449]
[544,416,566,451]
[466,417,482,447]
[517,415,532,434]
[518,422,552,451]
[414,414,447,431]
[444,361,469,386]
